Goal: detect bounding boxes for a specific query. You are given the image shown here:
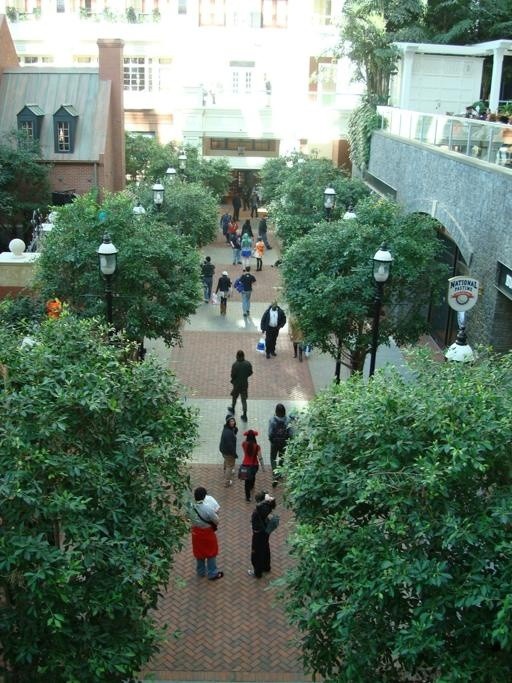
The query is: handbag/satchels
[265,514,279,534]
[208,520,217,531]
[238,464,252,481]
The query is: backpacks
[270,416,289,450]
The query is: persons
[288,314,306,362]
[454,106,510,166]
[239,265,257,317]
[220,414,238,488]
[227,350,253,422]
[219,172,272,272]
[200,256,216,303]
[268,403,296,487]
[215,271,233,316]
[240,429,267,502]
[260,299,286,359]
[183,487,224,581]
[248,493,279,578]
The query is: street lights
[298,157,306,174]
[342,205,359,245]
[134,202,144,222]
[443,325,475,365]
[178,150,188,174]
[322,181,336,223]
[151,181,165,215]
[96,228,121,333]
[16,333,41,354]
[166,164,176,191]
[366,240,395,386]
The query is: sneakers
[208,572,223,580]
[248,570,256,577]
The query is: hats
[226,415,235,423]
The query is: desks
[432,138,504,162]
